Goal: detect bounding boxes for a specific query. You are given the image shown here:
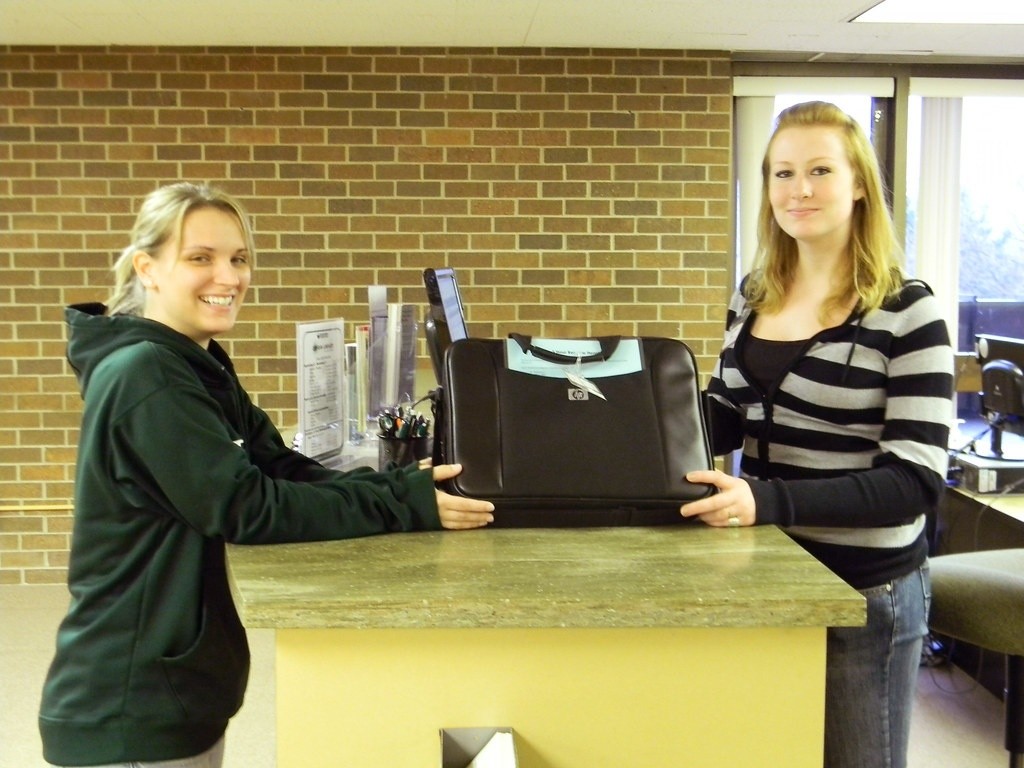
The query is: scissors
[378,414,405,438]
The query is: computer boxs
[947,454,1024,495]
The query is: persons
[679,100,954,768]
[37,181,495,768]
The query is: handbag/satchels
[439,333,717,524]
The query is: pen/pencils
[375,405,430,468]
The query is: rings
[728,517,740,526]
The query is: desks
[224,516,869,768]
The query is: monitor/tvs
[974,333,1024,462]
[424,266,468,385]
